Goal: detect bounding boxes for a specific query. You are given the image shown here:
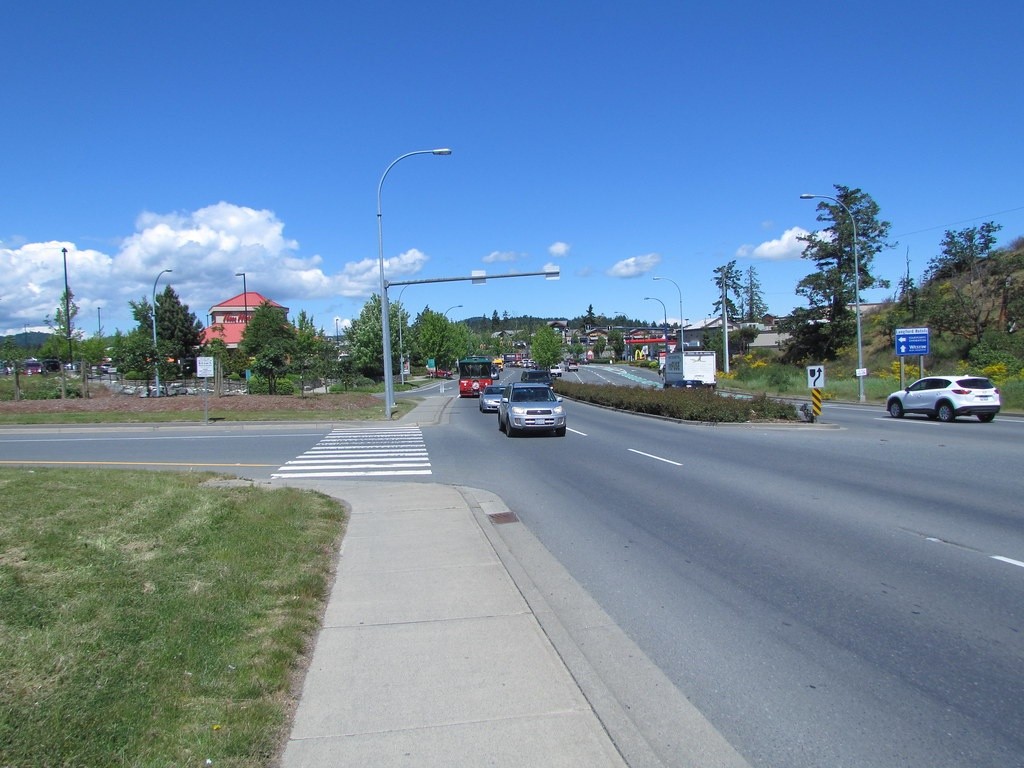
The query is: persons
[536,392,544,400]
[481,364,489,376]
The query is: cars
[427,369,453,379]
[511,359,536,369]
[493,358,504,380]
[0,357,82,377]
[564,357,590,365]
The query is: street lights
[645,297,668,351]
[444,305,462,317]
[799,194,867,405]
[653,277,684,352]
[97,305,101,341]
[61,247,74,369]
[23,322,29,360]
[235,273,247,327]
[151,269,173,396]
[378,149,451,416]
[336,318,338,349]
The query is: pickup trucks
[566,362,579,372]
[550,366,562,377]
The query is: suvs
[886,375,1002,422]
[91,363,118,374]
[478,370,568,437]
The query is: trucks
[661,352,718,390]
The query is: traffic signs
[894,327,930,356]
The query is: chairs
[924,381,937,389]
[513,391,521,400]
[487,389,494,395]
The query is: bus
[503,354,528,363]
[460,356,493,398]
